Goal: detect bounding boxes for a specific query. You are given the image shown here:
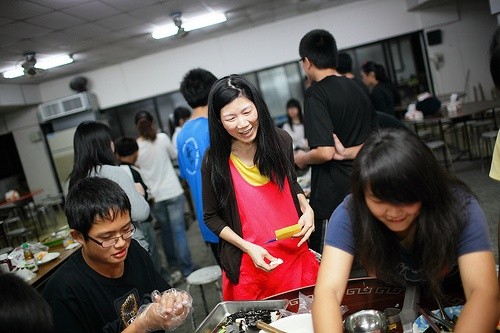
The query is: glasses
[85,222,136,248]
[298,58,304,63]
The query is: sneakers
[167,270,183,287]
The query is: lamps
[152,10,227,40]
[3,53,73,79]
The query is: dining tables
[194,277,499,333]
[413,98,500,168]
[0,225,83,297]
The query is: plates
[16,250,60,269]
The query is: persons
[113,136,182,287]
[202,74,321,302]
[64,121,152,255]
[360,61,393,116]
[298,30,377,255]
[132,109,202,281]
[488,23,500,180]
[281,99,309,153]
[0,271,56,333]
[172,106,194,152]
[310,129,500,333]
[180,68,221,270]
[41,176,193,333]
[335,52,370,99]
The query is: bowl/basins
[343,308,387,333]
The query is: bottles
[22,243,40,273]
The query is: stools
[425,140,449,169]
[479,130,500,168]
[0,192,66,255]
